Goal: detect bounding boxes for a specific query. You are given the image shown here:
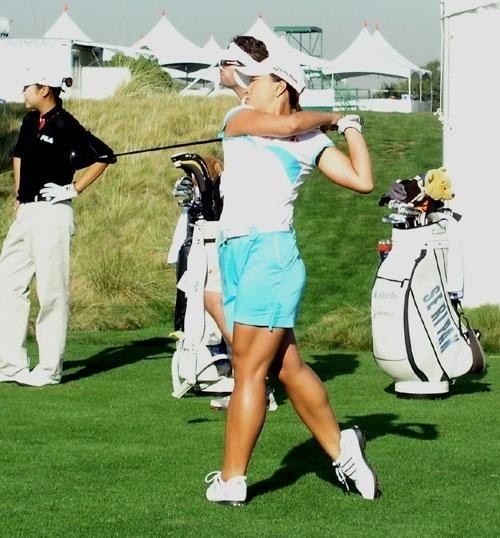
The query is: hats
[22,64,63,87]
[214,42,306,93]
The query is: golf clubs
[381,200,463,230]
[172,148,220,223]
[70,116,366,169]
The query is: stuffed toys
[388,165,456,230]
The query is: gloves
[40,183,79,204]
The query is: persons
[202,34,284,413]
[202,54,381,508]
[0,62,116,389]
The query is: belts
[23,194,71,202]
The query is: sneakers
[205,469,247,507]
[333,424,377,501]
[210,386,278,411]
[0,367,61,386]
